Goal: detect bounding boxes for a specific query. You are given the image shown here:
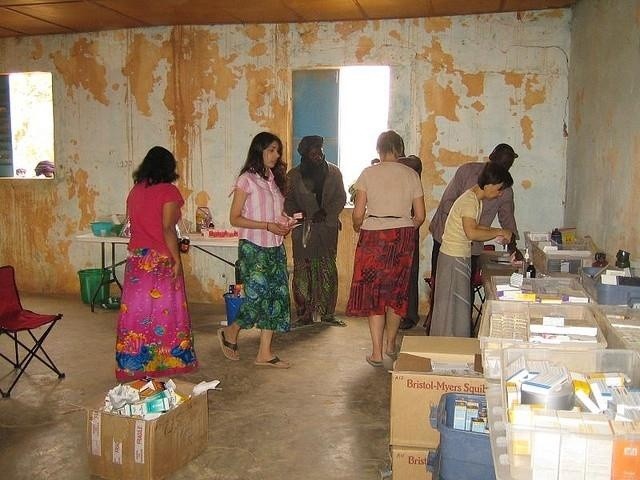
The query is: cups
[202,230,238,238]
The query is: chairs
[0,265,65,398]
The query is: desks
[77,232,239,312]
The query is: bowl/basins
[102,296,120,310]
[89,221,113,236]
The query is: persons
[115,144,199,382]
[34,160,54,177]
[425,143,523,336]
[216,131,299,370]
[344,129,427,368]
[370,138,421,331]
[286,135,347,329]
[428,162,514,337]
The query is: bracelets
[267,221,270,231]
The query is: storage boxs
[69,378,222,480]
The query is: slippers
[290,319,316,331]
[321,318,347,327]
[385,344,401,357]
[217,327,241,361]
[366,353,384,367]
[254,356,291,368]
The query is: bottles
[201,219,215,234]
[592,252,608,268]
[616,248,632,268]
[526,261,535,279]
[550,227,562,250]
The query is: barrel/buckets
[78,268,112,305]
[222,291,245,325]
